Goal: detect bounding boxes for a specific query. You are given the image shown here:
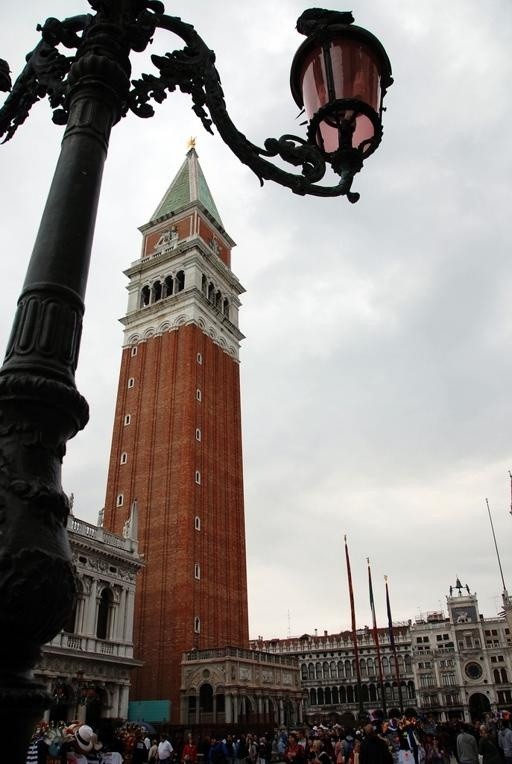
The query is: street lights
[2,0,395,762]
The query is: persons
[27,714,511,762]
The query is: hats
[75,724,94,752]
